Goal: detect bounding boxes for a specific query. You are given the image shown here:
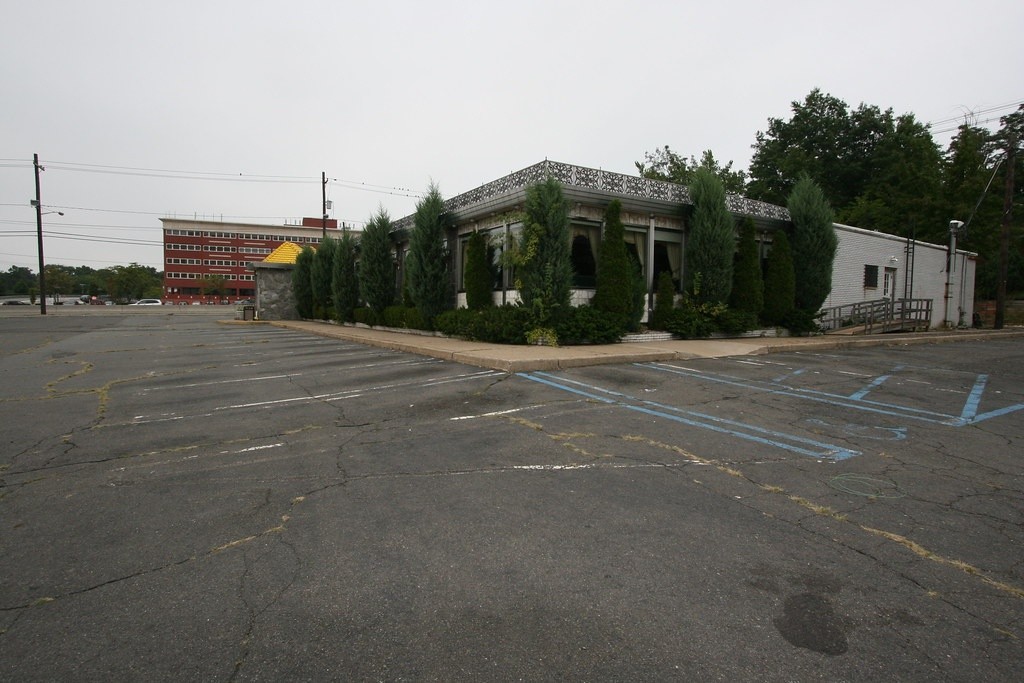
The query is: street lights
[946,220,965,327]
[37,212,65,312]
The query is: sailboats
[53,292,63,305]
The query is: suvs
[129,299,162,306]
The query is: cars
[233,299,254,304]
[206,302,215,306]
[179,301,187,305]
[165,301,174,305]
[221,299,229,305]
[192,300,202,305]
